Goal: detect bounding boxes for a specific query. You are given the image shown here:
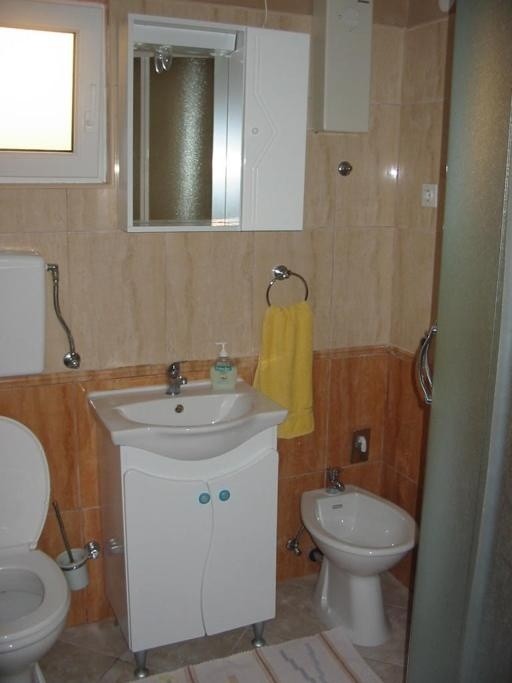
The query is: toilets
[0,250,73,683]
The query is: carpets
[129,624,383,683]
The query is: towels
[251,298,316,441]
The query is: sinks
[87,381,289,460]
[300,484,420,576]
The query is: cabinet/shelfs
[97,426,280,679]
[243,26,311,232]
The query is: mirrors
[115,12,247,233]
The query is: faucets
[164,361,187,395]
[325,464,345,494]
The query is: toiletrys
[209,342,238,390]
